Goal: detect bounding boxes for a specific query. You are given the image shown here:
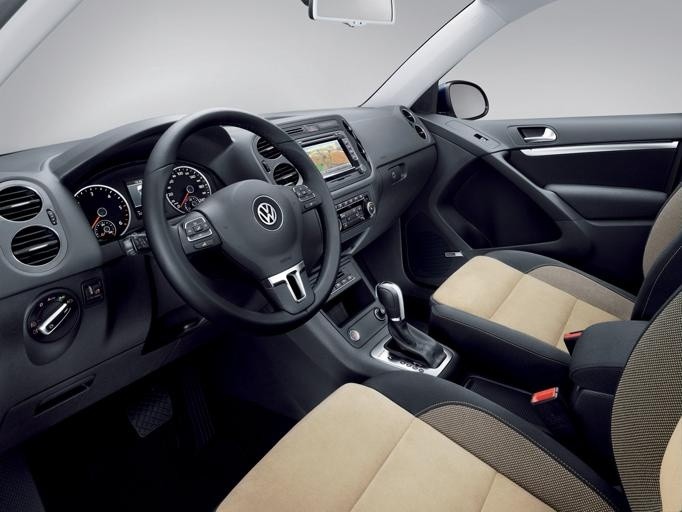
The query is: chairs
[432,178,682,382]
[201,276,682,512]
